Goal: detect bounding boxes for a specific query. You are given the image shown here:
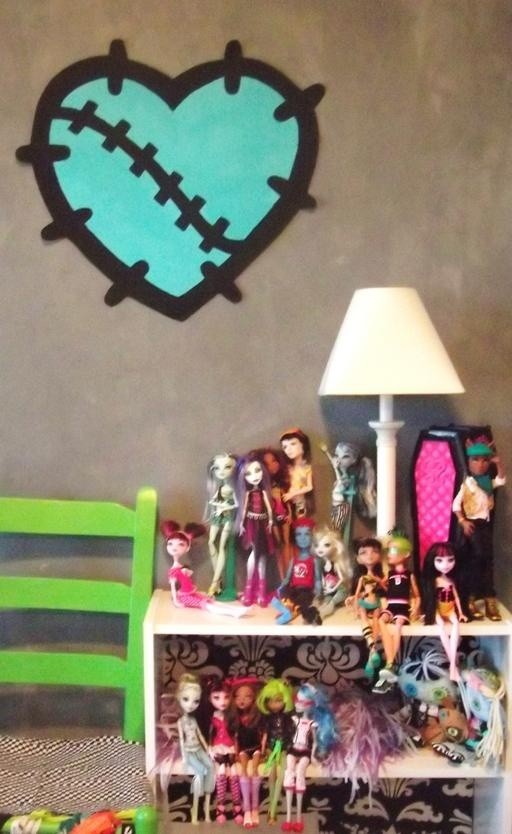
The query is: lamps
[315,286,469,577]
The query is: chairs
[1,486,172,834]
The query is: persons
[158,427,512,694]
[174,668,512,830]
[160,428,511,831]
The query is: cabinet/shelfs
[142,589,511,832]
